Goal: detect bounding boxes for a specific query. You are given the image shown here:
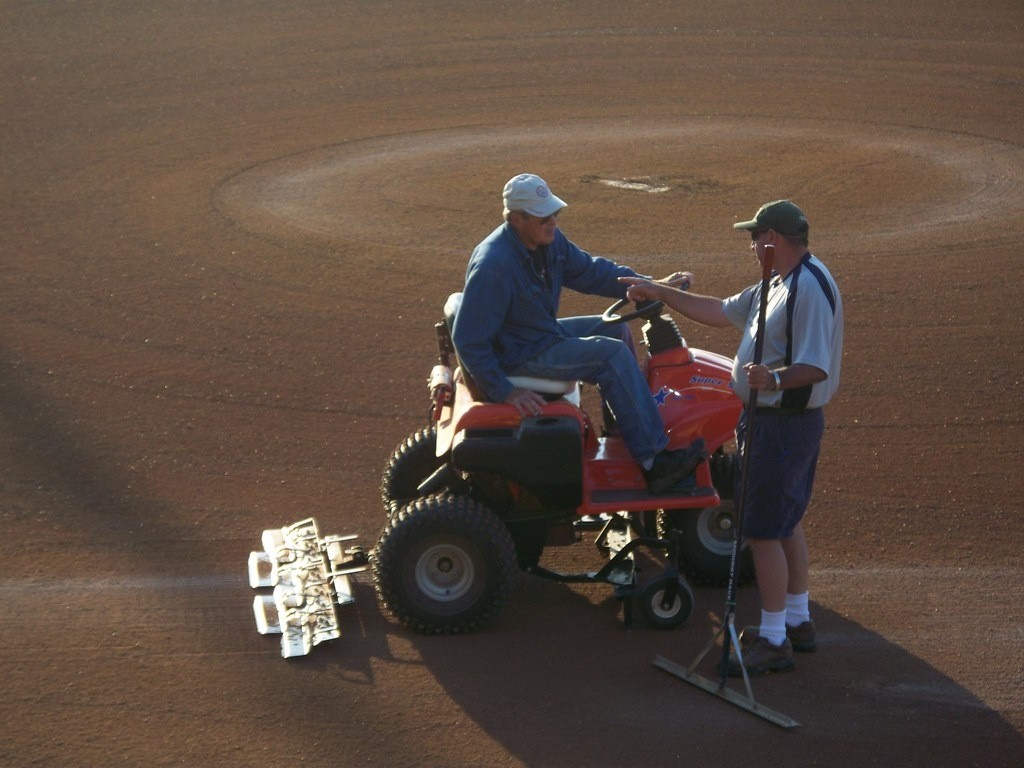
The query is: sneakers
[645,438,710,498]
[742,618,820,654]
[605,420,623,437]
[716,636,798,678]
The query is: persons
[617,198,843,677]
[452,173,707,495]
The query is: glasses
[522,209,563,226]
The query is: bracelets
[770,370,780,392]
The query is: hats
[734,198,810,236]
[502,172,568,217]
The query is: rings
[525,404,531,409]
